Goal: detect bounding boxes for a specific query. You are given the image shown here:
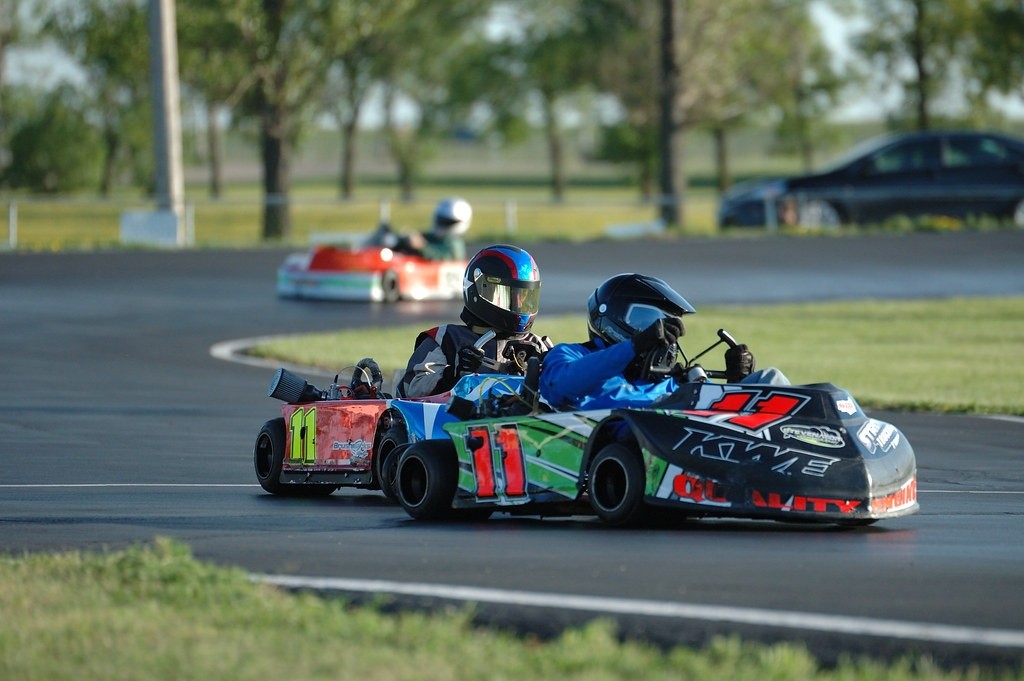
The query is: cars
[716,124,1023,232]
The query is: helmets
[459,243,541,333]
[586,272,697,351]
[431,196,473,237]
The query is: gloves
[631,317,686,354]
[457,344,484,377]
[725,343,755,383]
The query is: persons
[536,272,756,414]
[396,244,548,399]
[408,198,472,260]
